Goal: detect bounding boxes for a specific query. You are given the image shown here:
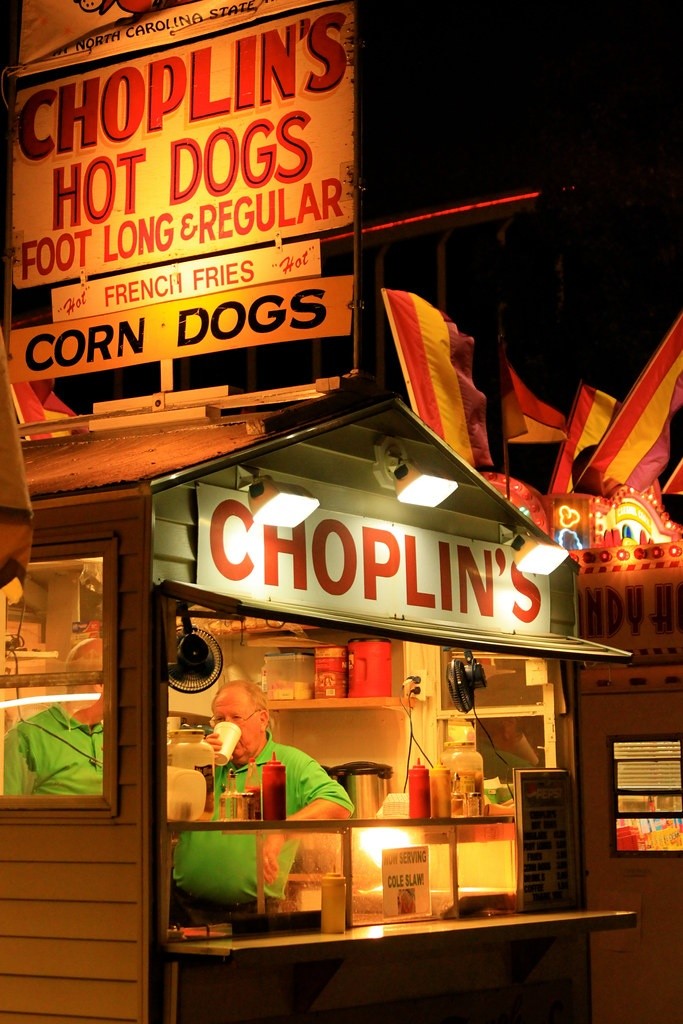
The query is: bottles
[262,751,286,820]
[167,724,215,822]
[320,864,346,933]
[242,793,254,820]
[409,759,431,818]
[430,756,452,818]
[452,773,466,817]
[245,758,261,820]
[347,637,392,698]
[441,742,485,817]
[219,768,243,820]
[477,792,485,817]
[466,792,479,816]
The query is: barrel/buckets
[315,646,353,699]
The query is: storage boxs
[262,649,317,702]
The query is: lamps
[388,445,457,510]
[500,522,569,577]
[237,465,320,530]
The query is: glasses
[209,709,258,727]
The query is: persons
[3,638,104,796]
[172,681,354,928]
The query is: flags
[591,309,683,492]
[499,344,569,443]
[384,288,493,467]
[552,384,618,497]
[9,377,90,441]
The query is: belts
[174,888,256,916]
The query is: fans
[444,648,487,716]
[166,601,225,695]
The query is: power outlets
[411,670,427,701]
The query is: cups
[213,723,242,766]
